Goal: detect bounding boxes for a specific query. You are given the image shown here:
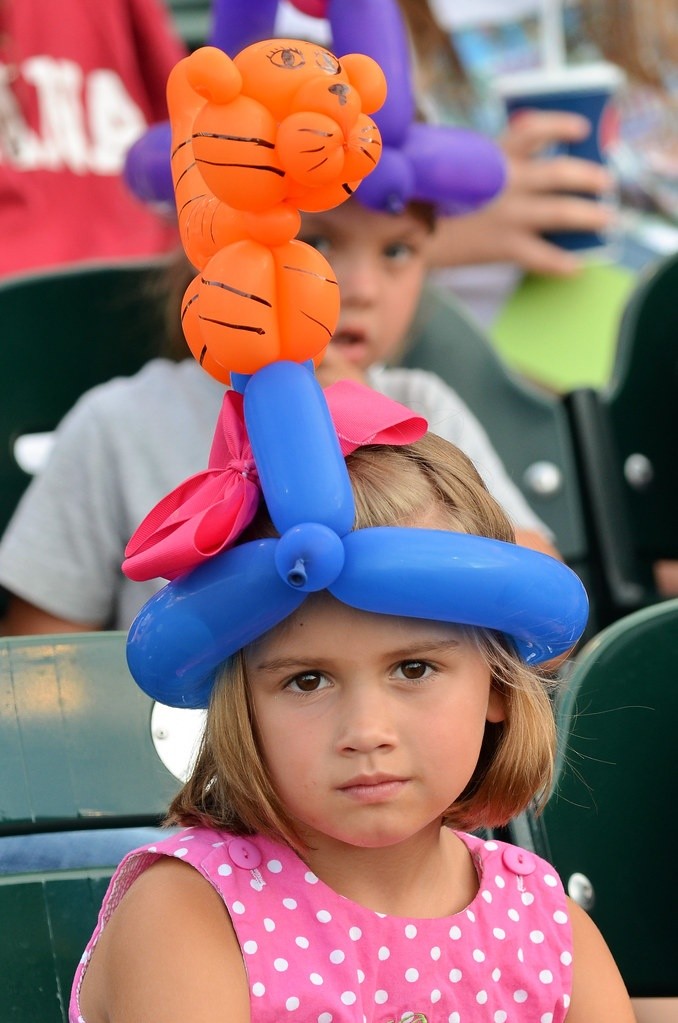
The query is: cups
[499,65,618,251]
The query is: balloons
[122,1,587,710]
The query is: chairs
[0,259,582,635]
[563,253,677,618]
[509,597,678,998]
[0,825,494,1023]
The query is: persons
[66,423,634,1023]
[272,0,678,406]
[0,189,560,638]
